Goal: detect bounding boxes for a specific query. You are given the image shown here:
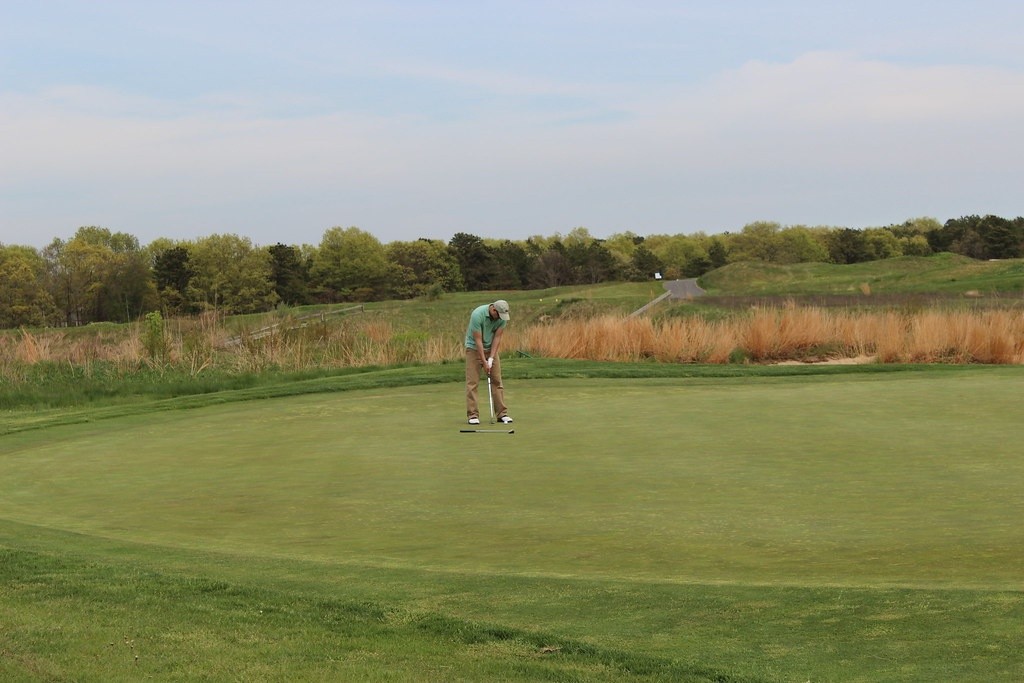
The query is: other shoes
[497,416,513,422]
[468,418,480,424]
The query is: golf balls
[504,420,508,424]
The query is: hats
[494,300,510,320]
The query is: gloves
[488,358,494,368]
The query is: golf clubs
[460,429,515,434]
[487,370,497,424]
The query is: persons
[465,301,514,423]
